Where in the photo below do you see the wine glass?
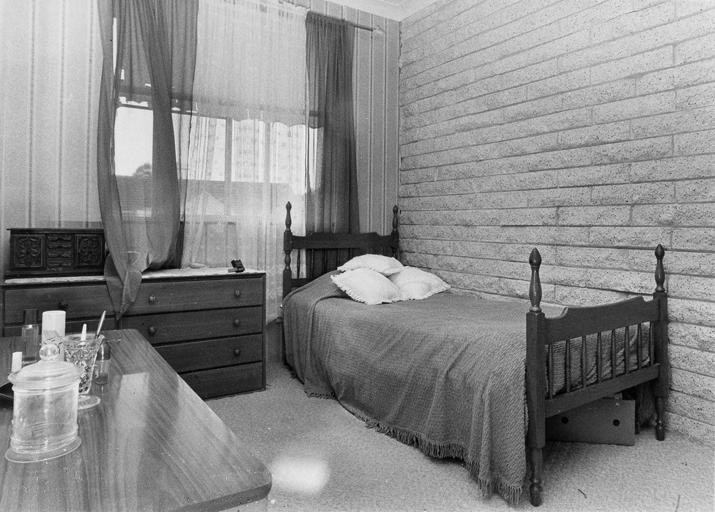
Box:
[61,332,103,411]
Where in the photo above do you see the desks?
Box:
[0,330,273,512]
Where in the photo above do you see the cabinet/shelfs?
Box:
[3,266,273,402]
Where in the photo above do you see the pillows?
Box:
[331,253,450,306]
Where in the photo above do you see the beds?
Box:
[281,199,672,508]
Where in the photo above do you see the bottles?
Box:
[3,343,83,464]
[40,309,68,360]
[93,338,111,385]
[20,307,41,364]
